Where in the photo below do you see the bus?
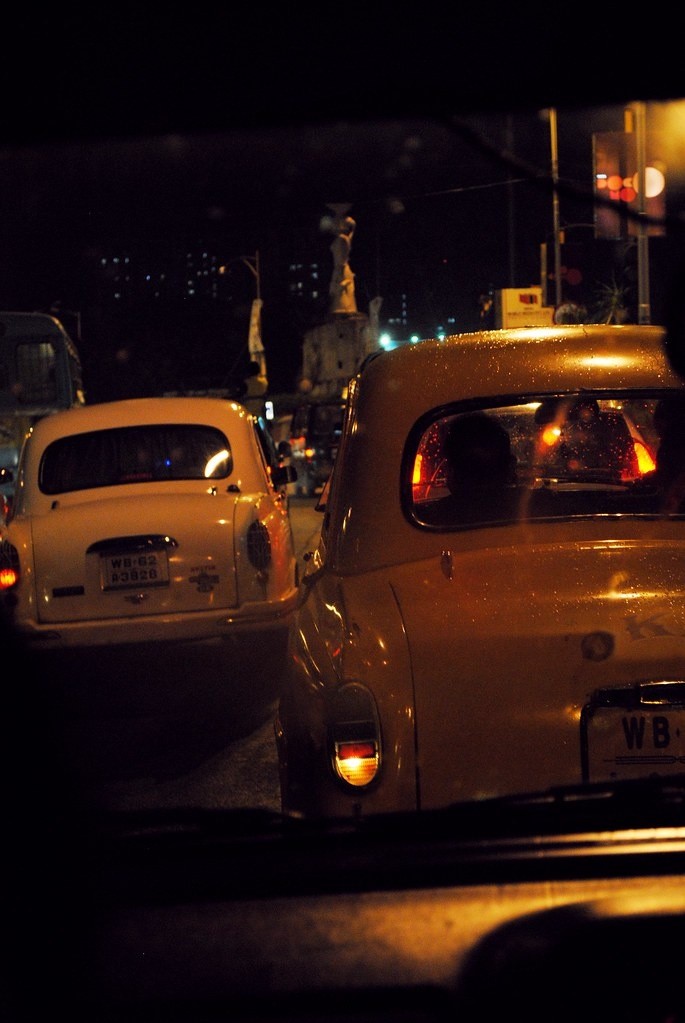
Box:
[0,312,85,479]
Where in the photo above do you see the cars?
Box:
[412,403,661,499]
[279,322,683,812]
[0,400,306,655]
[284,400,350,494]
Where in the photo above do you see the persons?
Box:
[73,436,208,476]
[427,413,545,517]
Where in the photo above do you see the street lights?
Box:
[539,106,566,311]
[217,250,267,378]
